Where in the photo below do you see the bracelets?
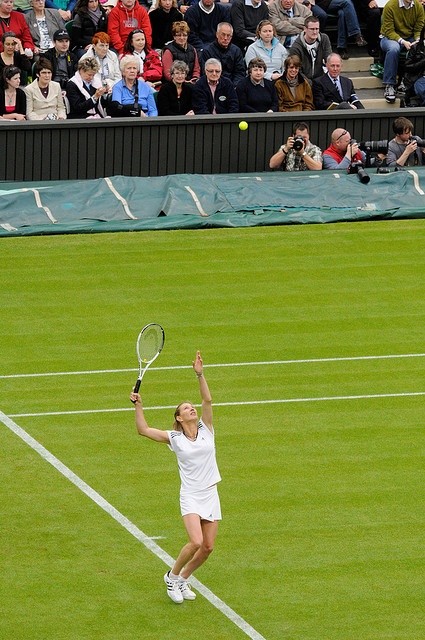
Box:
[195,373,203,377]
[282,148,288,155]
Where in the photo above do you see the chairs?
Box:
[63,21,74,28]
[31,63,38,75]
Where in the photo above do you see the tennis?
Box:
[239,121,248,130]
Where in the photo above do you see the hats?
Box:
[54,29,70,40]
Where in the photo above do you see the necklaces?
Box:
[5,89,16,106]
[39,84,49,94]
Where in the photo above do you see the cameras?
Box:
[409,135,425,148]
[347,162,371,184]
[293,135,305,155]
[346,139,389,156]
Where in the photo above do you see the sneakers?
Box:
[339,48,349,59]
[397,84,407,94]
[176,580,197,601]
[354,36,368,47]
[384,86,396,103]
[163,570,185,603]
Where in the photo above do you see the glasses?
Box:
[337,129,349,140]
[306,27,319,30]
[175,34,187,37]
[40,71,53,74]
[173,72,186,75]
[206,68,221,73]
[132,28,144,33]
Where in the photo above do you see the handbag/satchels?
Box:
[121,102,143,118]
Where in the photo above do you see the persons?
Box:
[45,0,79,22]
[25,57,67,120]
[298,0,329,32]
[380,0,425,104]
[157,59,198,115]
[119,29,163,81]
[386,117,425,167]
[268,122,323,172]
[317,1,368,60]
[136,0,157,13]
[97,0,120,16]
[0,65,27,120]
[71,0,119,58]
[162,21,201,85]
[274,55,316,111]
[404,26,425,107]
[353,0,381,59]
[232,0,269,45]
[288,15,333,80]
[178,0,199,15]
[184,1,232,53]
[65,59,113,118]
[243,19,289,81]
[313,52,361,109]
[107,0,153,59]
[237,58,279,112]
[322,127,366,170]
[269,0,313,36]
[0,0,35,60]
[40,30,78,88]
[199,22,246,89]
[192,57,240,113]
[25,0,68,55]
[78,31,124,100]
[128,350,222,606]
[148,0,184,51]
[0,31,31,86]
[111,54,158,116]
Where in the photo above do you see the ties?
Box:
[286,11,291,47]
[334,79,340,96]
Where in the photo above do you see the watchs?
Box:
[299,152,307,158]
